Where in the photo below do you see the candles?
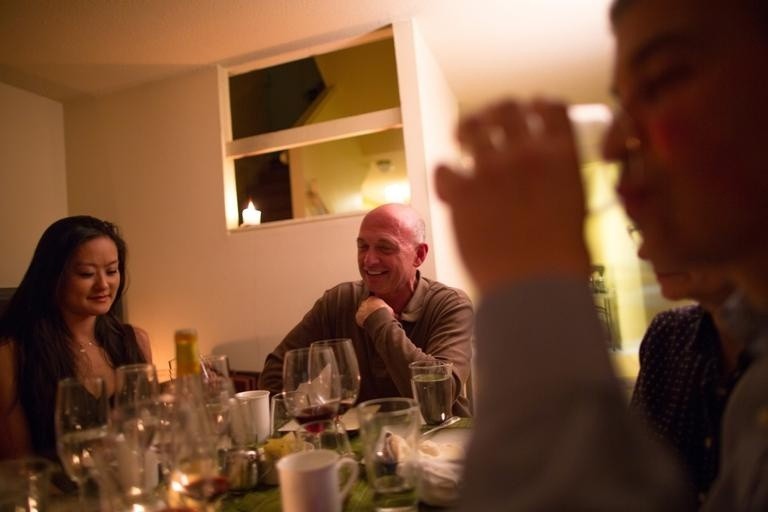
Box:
[240,200,260,224]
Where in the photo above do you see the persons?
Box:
[0,215,157,498]
[256,201,476,421]
[433,0,768,510]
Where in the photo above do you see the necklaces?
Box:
[64,332,99,353]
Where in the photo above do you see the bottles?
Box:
[171,327,209,414]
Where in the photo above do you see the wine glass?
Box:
[54,337,362,511]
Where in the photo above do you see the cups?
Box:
[354,397,422,511]
[434,95,646,218]
[409,358,454,425]
[1,456,54,512]
